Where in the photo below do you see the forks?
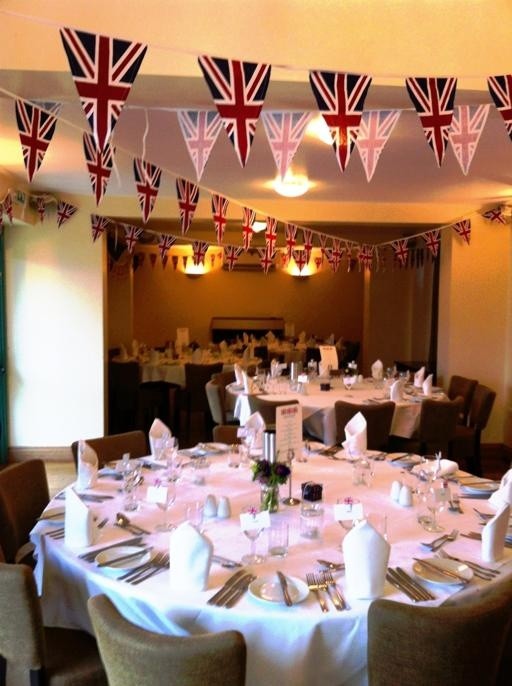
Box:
[118,553,171,584]
[305,569,346,613]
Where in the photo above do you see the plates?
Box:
[247,576,309,604]
[95,546,150,569]
[413,559,474,586]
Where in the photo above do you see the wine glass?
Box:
[38,426,512,561]
[251,359,412,399]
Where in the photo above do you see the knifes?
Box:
[207,568,255,610]
[412,558,467,584]
[276,571,294,607]
[386,566,432,604]
